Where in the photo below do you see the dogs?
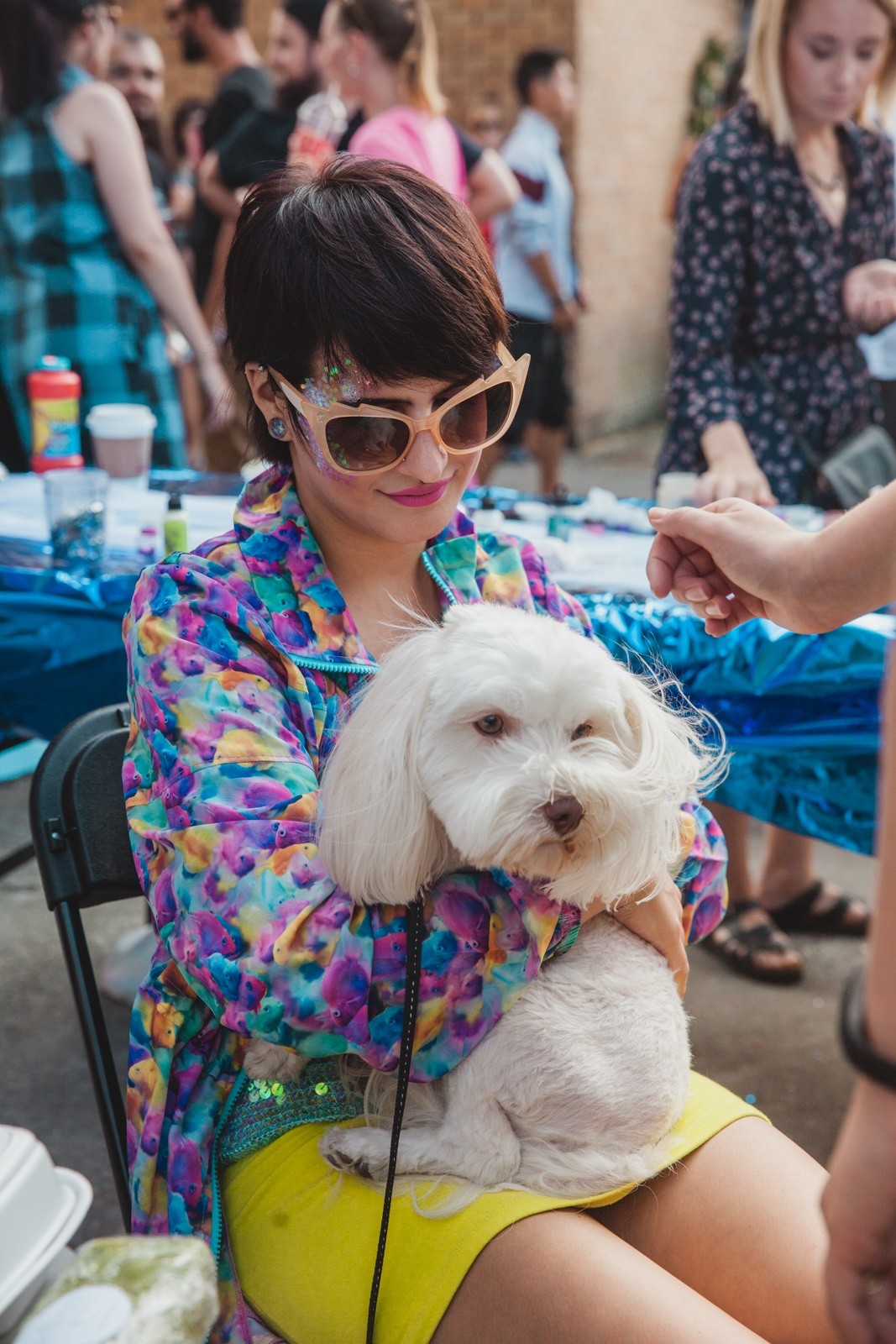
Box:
[302,587,730,1221]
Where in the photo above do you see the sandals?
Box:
[771,876,871,938]
[697,896,802,986]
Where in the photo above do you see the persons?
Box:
[0,0,896,1344]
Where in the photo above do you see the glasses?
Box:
[262,334,532,478]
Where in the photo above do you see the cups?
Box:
[43,467,111,583]
[85,403,157,480]
[286,91,348,183]
[657,472,696,509]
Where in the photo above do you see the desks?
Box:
[0,467,896,883]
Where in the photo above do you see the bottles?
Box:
[29,353,83,474]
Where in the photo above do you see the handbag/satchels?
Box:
[820,422,896,511]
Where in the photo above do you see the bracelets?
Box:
[834,962,896,1094]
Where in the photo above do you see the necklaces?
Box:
[807,167,842,194]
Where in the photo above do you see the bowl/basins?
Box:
[0,1121,93,1336]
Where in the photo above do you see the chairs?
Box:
[26,700,151,1224]
